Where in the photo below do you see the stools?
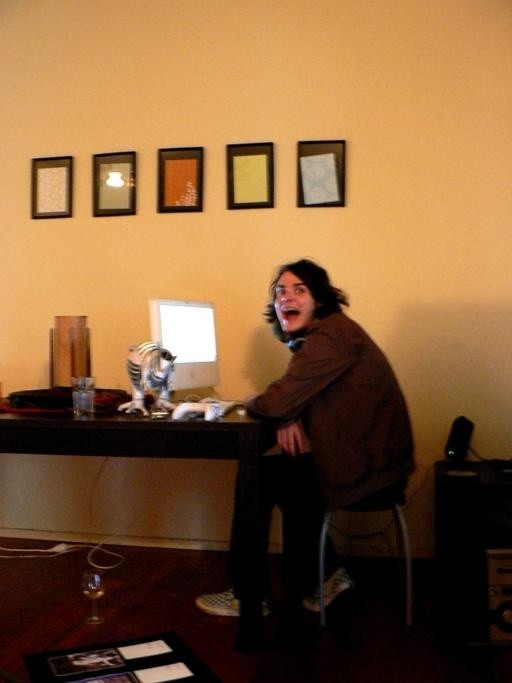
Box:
[313,493,414,629]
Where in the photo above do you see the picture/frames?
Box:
[226,142,275,211]
[157,146,204,213]
[296,139,346,208]
[92,150,137,217]
[31,155,73,221]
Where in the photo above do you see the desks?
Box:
[0,387,310,556]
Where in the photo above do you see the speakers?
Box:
[444,415,475,459]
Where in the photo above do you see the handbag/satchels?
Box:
[0,386,127,415]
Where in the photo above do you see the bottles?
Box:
[72,377,96,420]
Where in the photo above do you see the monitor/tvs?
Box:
[149,299,220,410]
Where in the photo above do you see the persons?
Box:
[197,260,415,617]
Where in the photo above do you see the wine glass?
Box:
[81,569,105,625]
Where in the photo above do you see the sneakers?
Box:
[196,588,269,617]
[304,567,354,611]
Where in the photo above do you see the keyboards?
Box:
[198,397,237,417]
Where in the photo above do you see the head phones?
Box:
[288,300,335,353]
